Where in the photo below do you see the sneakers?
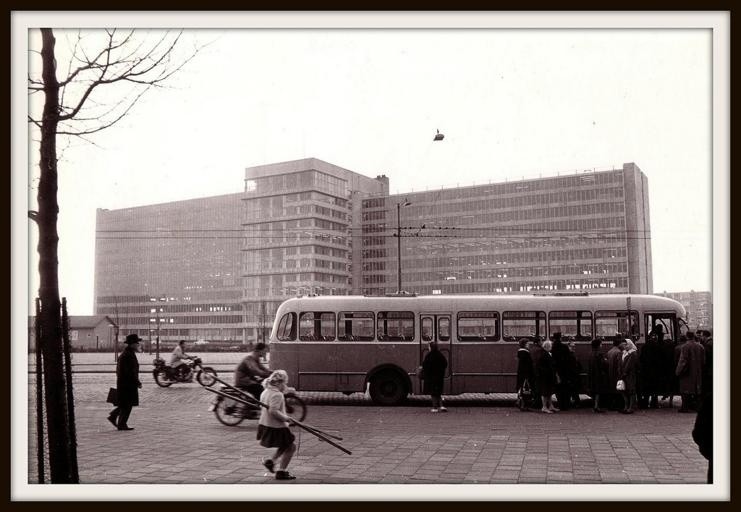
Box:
[264,460,275,473]
[430,406,447,412]
[106,415,134,430]
[540,404,559,414]
[276,471,296,480]
[592,404,632,414]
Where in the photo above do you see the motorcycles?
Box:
[206,380,308,427]
[150,355,219,388]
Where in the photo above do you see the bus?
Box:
[260,295,700,408]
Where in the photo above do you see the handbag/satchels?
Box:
[518,380,534,409]
[616,379,625,390]
[107,387,118,406]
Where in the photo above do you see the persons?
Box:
[255,370,297,480]
[421,341,448,413]
[234,342,272,402]
[692,392,712,483]
[107,332,143,431]
[516,324,712,414]
[169,340,193,368]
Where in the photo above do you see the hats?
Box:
[124,334,142,343]
